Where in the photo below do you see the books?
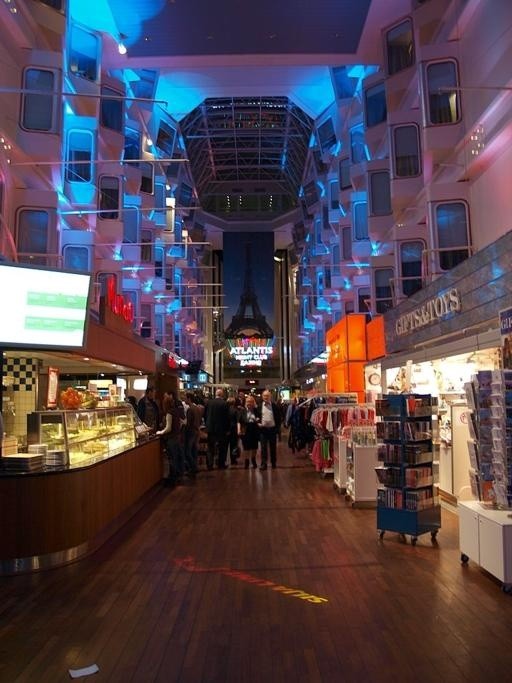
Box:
[462,367,511,505]
[373,393,442,514]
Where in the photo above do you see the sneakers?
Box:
[185,473,196,480]
[176,478,182,483]
[191,468,198,472]
[164,481,174,487]
[206,458,277,470]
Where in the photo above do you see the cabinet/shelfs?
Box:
[439,445,452,494]
[377,394,442,547]
[25,403,136,465]
[457,370,512,592]
[346,438,384,509]
[289,392,377,476]
[450,402,472,494]
[332,434,347,494]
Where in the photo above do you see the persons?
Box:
[124,385,317,485]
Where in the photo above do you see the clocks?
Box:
[369,373,381,384]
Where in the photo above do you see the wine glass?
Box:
[110,395,120,408]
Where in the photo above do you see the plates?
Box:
[53,434,79,441]
[46,450,68,470]
[27,445,51,470]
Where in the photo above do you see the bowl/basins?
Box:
[57,399,84,411]
[82,398,100,411]
